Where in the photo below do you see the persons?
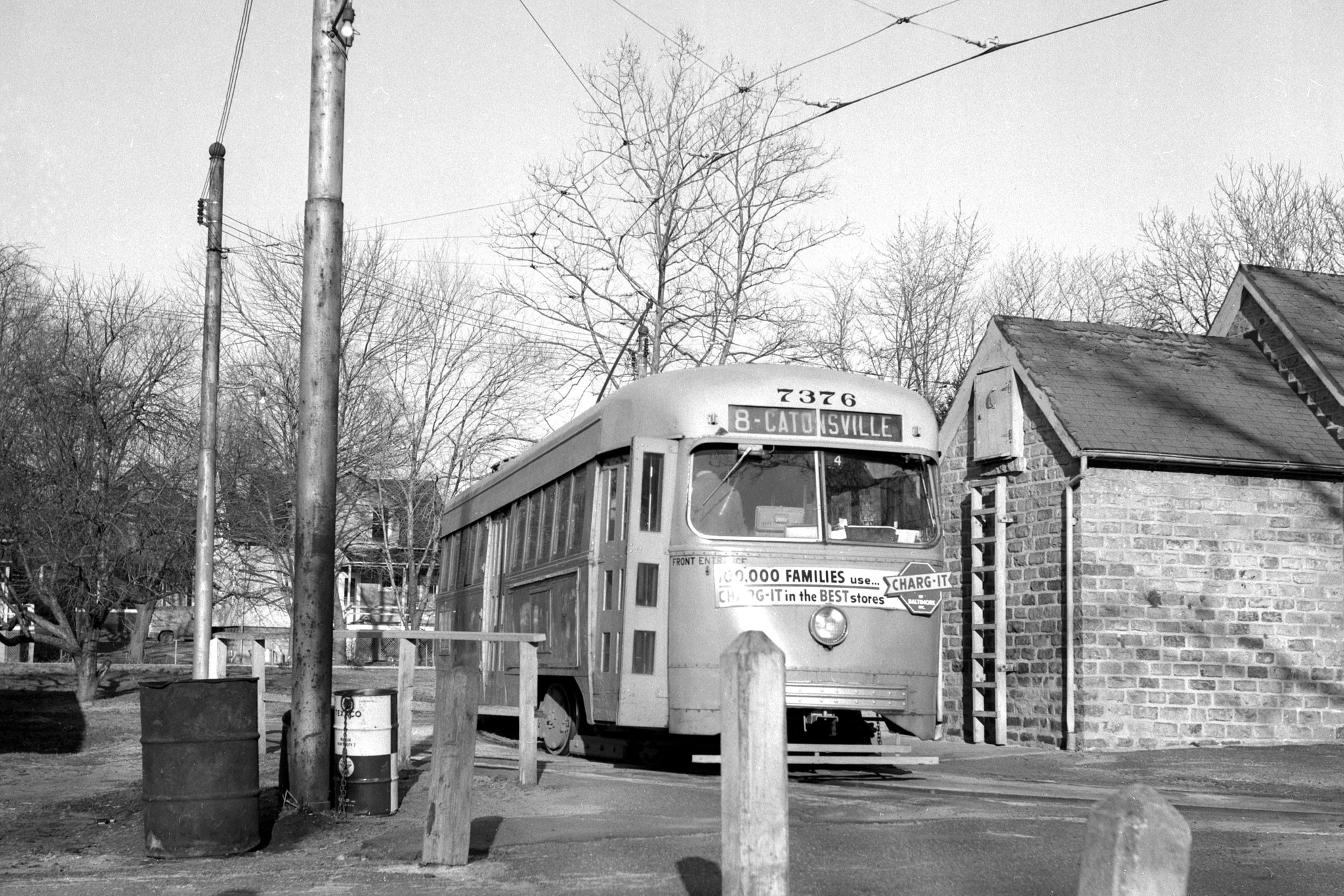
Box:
[689,451,747,539]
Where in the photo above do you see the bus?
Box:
[440,362,947,780]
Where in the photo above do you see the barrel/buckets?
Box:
[139,674,263,858]
[335,686,401,816]
[278,703,336,807]
